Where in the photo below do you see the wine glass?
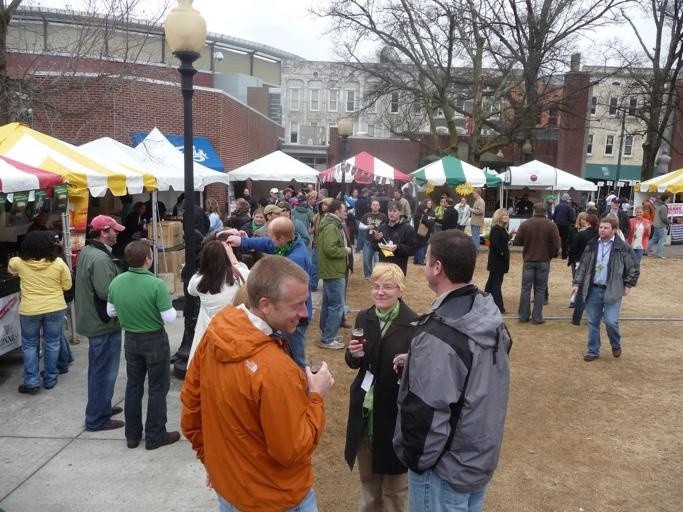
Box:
[310,359,321,374]
[352,328,363,357]
[394,354,406,384]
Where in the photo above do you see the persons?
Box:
[391,228,514,512]
[179,254,336,512]
[342,260,420,512]
[187,175,518,370]
[515,190,671,362]
[7,193,184,451]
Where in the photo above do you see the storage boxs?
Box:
[158,250,185,292]
[148,220,184,249]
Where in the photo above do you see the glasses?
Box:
[371,285,400,290]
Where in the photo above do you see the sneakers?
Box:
[320,341,345,349]
[612,347,622,358]
[584,352,599,361]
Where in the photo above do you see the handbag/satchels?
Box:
[417,215,428,237]
[665,223,671,246]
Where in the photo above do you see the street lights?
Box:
[521,137,534,163]
[337,118,354,199]
[162,0,208,379]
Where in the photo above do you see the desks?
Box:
[465,217,528,241]
[0,290,21,356]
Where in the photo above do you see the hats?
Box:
[612,198,621,203]
[270,188,278,193]
[90,214,126,232]
[264,205,286,215]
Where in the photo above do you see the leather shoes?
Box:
[19,384,40,393]
[109,407,123,415]
[145,431,180,449]
[105,420,124,430]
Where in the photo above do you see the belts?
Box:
[593,284,607,288]
